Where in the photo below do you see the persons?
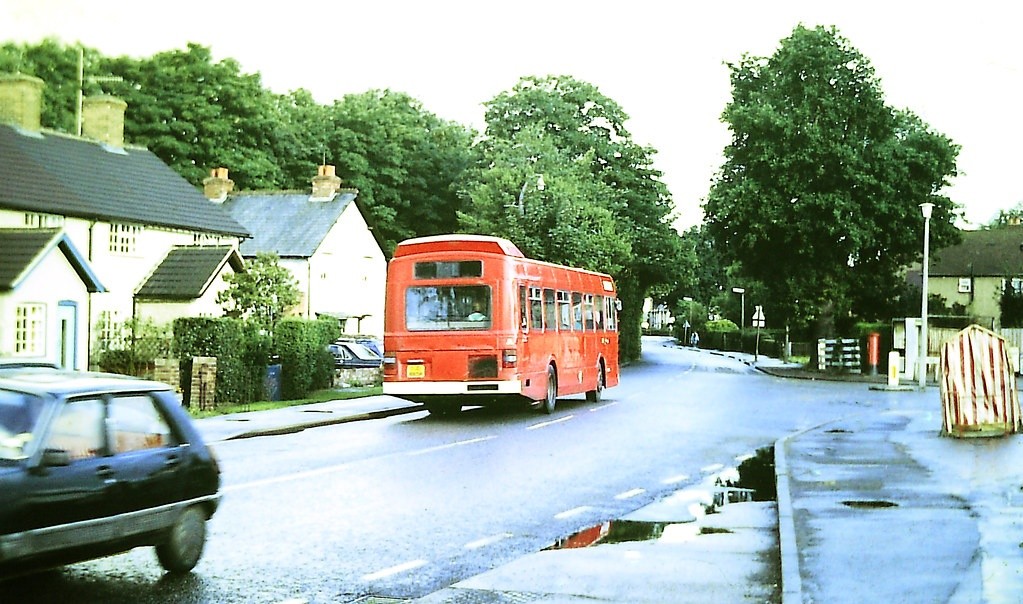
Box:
[690,331,699,348]
[468,303,487,321]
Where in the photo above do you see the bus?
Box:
[382,234,623,415]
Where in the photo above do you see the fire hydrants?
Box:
[867,332,880,375]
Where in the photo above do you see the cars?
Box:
[326,342,383,369]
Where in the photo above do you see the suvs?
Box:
[0,363,223,572]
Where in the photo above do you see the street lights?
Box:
[733,287,745,350]
[519,174,544,216]
[918,203,935,388]
[683,297,692,344]
